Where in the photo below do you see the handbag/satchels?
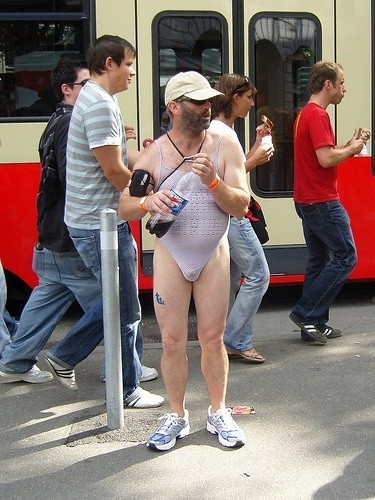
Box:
[251,217,269,244]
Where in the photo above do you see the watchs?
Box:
[139,196,148,212]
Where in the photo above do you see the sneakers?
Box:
[0,363,53,383]
[288,312,327,344]
[206,405,246,448]
[146,409,191,451]
[103,386,165,408]
[301,324,342,341]
[44,355,79,392]
[100,363,158,382]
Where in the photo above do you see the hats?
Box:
[165,71,225,107]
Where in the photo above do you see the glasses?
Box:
[174,97,214,105]
[232,76,250,94]
[66,79,88,87]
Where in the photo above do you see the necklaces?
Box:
[166,130,206,159]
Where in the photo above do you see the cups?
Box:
[356,128,370,142]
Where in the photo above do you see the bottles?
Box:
[144,172,201,238]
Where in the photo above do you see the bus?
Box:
[0,0,375,294]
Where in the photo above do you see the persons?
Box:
[43,35,163,408]
[0,60,157,383]
[119,70,250,450]
[0,259,19,360]
[205,74,274,363]
[288,61,369,343]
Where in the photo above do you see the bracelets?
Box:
[206,174,220,190]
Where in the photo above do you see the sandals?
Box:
[225,345,265,362]
